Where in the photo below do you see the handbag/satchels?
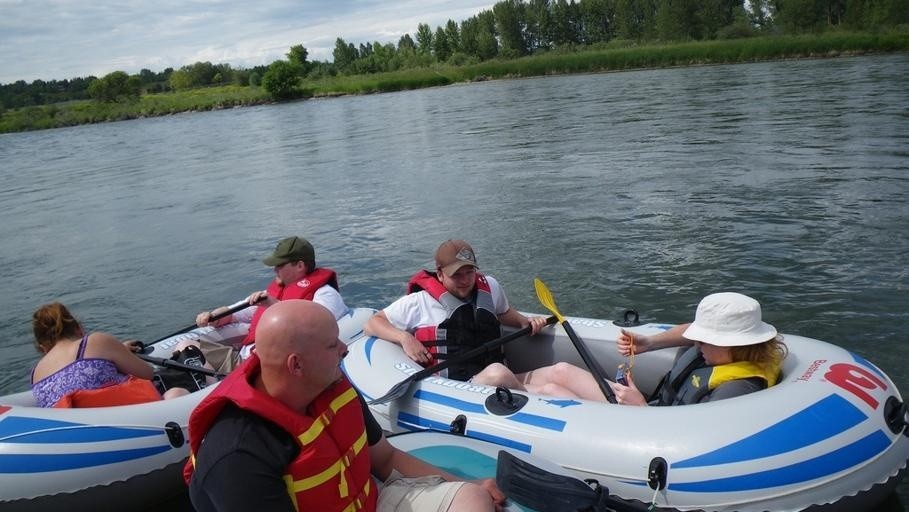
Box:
[152,345,205,394]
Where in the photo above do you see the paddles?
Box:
[365,315,559,404]
[533,278,617,404]
[130,294,268,351]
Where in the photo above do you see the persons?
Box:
[514,292,788,407]
[176,235,348,387]
[363,238,547,392]
[183,298,507,512]
[29,302,192,410]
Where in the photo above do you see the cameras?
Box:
[617,362,631,386]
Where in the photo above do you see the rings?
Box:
[424,351,429,356]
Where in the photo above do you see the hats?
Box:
[263,236,314,266]
[682,292,777,346]
[436,240,479,277]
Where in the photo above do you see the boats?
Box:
[341,292,899,510]
[3,296,377,511]
